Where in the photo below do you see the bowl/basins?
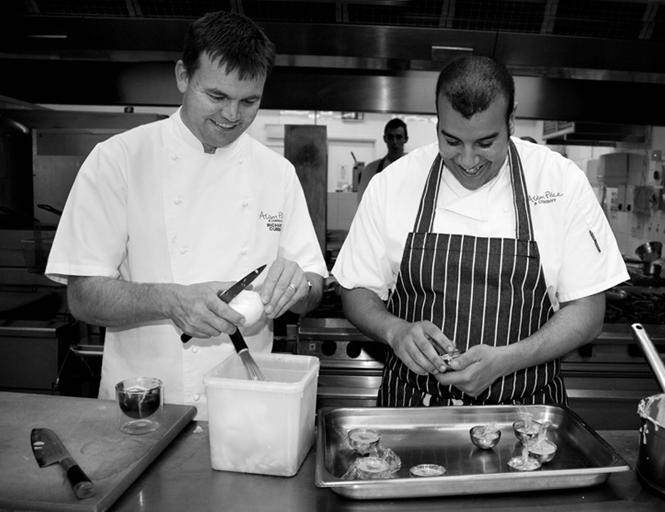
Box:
[643,264,662,275]
[635,241,663,263]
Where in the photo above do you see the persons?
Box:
[356,117,409,207]
[43,5,332,423]
[328,50,631,410]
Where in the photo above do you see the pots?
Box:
[628,320,665,500]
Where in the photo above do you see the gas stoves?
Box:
[562,288,665,360]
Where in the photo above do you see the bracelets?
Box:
[307,277,314,295]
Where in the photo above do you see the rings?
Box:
[288,282,300,294]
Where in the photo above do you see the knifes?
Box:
[29,427,96,501]
[180,265,267,345]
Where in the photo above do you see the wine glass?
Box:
[114,378,164,435]
